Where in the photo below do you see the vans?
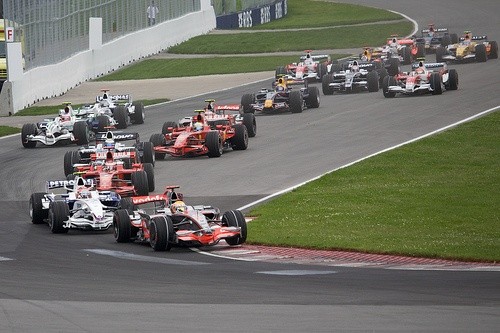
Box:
[0,20,27,82]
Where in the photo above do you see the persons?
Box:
[361,56,368,62]
[193,122,203,131]
[171,201,188,214]
[462,40,470,46]
[390,43,397,48]
[428,32,434,37]
[304,60,312,65]
[275,84,285,92]
[205,110,214,118]
[351,66,359,72]
[61,113,71,121]
[73,190,92,213]
[415,68,424,74]
[103,139,115,149]
[101,99,110,107]
[104,160,117,173]
[146,0,159,26]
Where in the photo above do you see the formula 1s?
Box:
[114,185,249,253]
[275,22,459,96]
[20,87,257,233]
[382,58,459,98]
[434,29,498,65]
[240,74,321,114]
